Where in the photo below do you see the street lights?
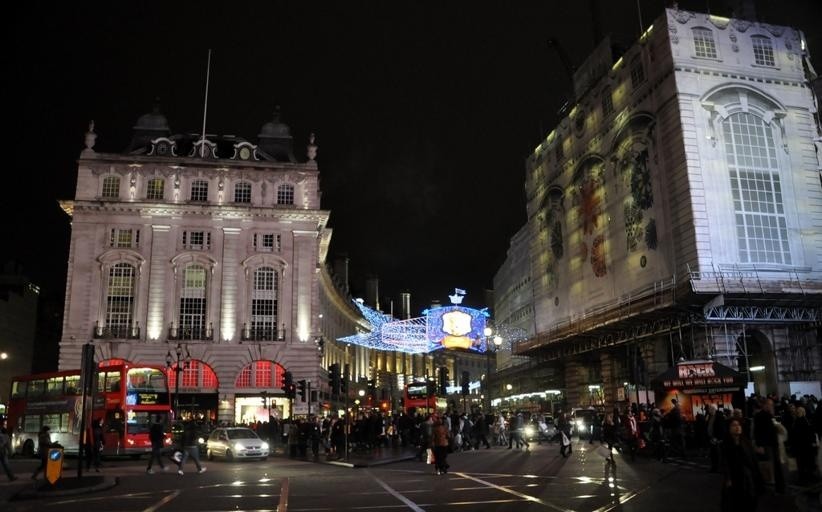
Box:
[476,319,503,415]
[166,343,191,419]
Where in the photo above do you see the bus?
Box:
[404,382,436,417]
[6,363,173,460]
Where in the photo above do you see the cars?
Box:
[171,419,216,453]
[205,426,270,463]
[485,407,604,443]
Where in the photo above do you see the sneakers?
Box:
[177,469,185,475]
[433,466,447,476]
[198,466,207,474]
[160,464,169,473]
[145,468,155,475]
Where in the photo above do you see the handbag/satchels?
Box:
[169,449,183,465]
[426,447,437,465]
[96,441,104,452]
[438,438,449,446]
[596,445,612,457]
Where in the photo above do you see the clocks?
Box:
[157,143,169,155]
[199,145,210,157]
[239,147,250,160]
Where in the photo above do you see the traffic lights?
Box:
[297,379,306,402]
[463,371,470,396]
[441,367,449,395]
[260,390,267,408]
[341,375,348,392]
[288,385,296,398]
[281,371,291,397]
[327,362,340,395]
[367,379,376,399]
[429,380,436,398]
[271,399,276,409]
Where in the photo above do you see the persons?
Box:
[430,419,450,475]
[145,415,202,475]
[0,426,19,482]
[253,413,576,457]
[86,417,104,473]
[31,426,51,481]
[601,394,822,489]
